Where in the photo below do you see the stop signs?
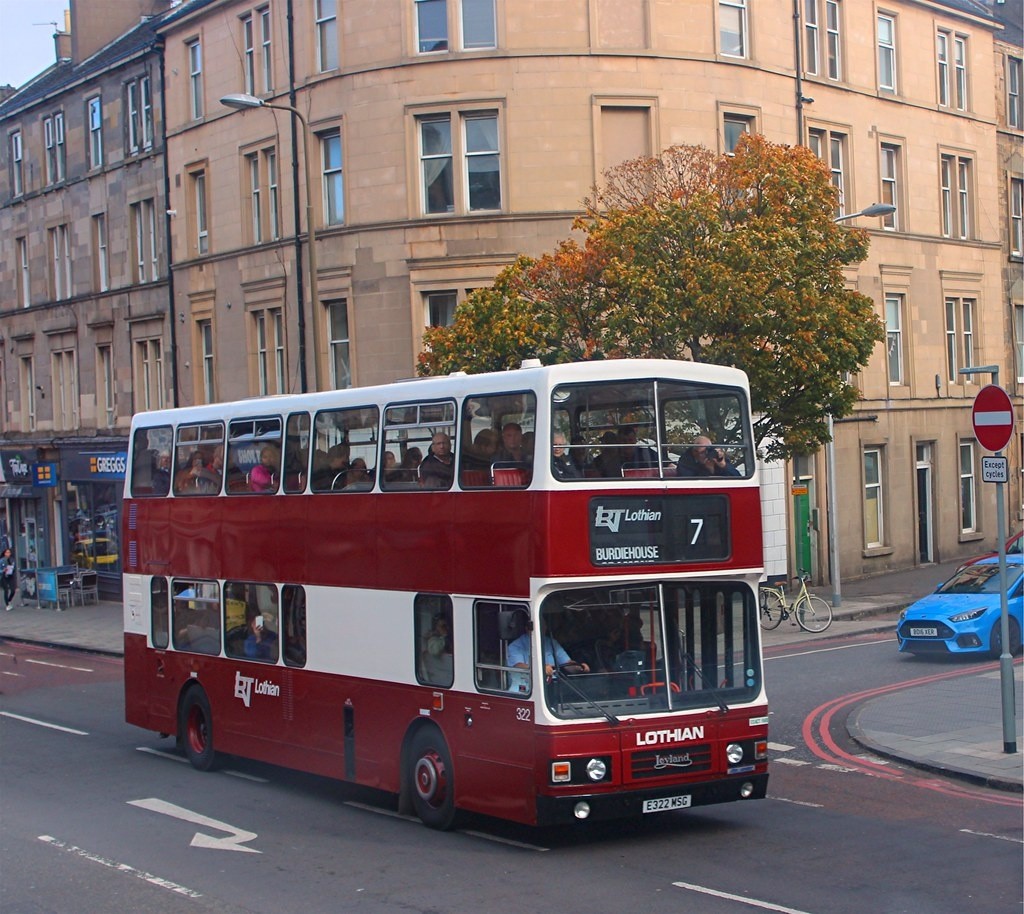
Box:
[970,385,1015,454]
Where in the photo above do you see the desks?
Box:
[20,567,76,576]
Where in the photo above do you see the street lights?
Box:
[219,93,324,393]
[826,204,896,606]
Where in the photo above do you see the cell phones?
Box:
[196,459,202,466]
[256,616,264,630]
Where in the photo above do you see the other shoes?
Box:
[8,602,14,609]
[6,606,11,611]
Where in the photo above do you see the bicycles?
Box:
[759,568,834,633]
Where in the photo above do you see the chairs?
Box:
[35,569,100,611]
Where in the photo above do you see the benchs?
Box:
[583,461,607,477]
[621,460,679,477]
[491,460,533,486]
[227,474,251,492]
[246,473,252,492]
[331,468,419,491]
[271,470,300,490]
[417,464,490,487]
[298,471,306,490]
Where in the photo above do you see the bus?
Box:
[121,359,769,830]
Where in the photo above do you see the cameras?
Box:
[705,447,723,462]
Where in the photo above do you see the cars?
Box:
[896,531,1024,659]
[68,504,119,570]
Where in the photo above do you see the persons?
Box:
[676,436,742,476]
[386,448,422,483]
[488,423,534,470]
[469,428,497,469]
[0,549,17,611]
[553,433,579,479]
[242,617,278,662]
[426,613,452,685]
[591,432,616,478]
[549,610,585,658]
[606,427,677,478]
[506,619,590,696]
[205,445,233,476]
[419,432,454,487]
[604,625,624,662]
[249,443,369,494]
[368,452,395,482]
[175,451,219,494]
